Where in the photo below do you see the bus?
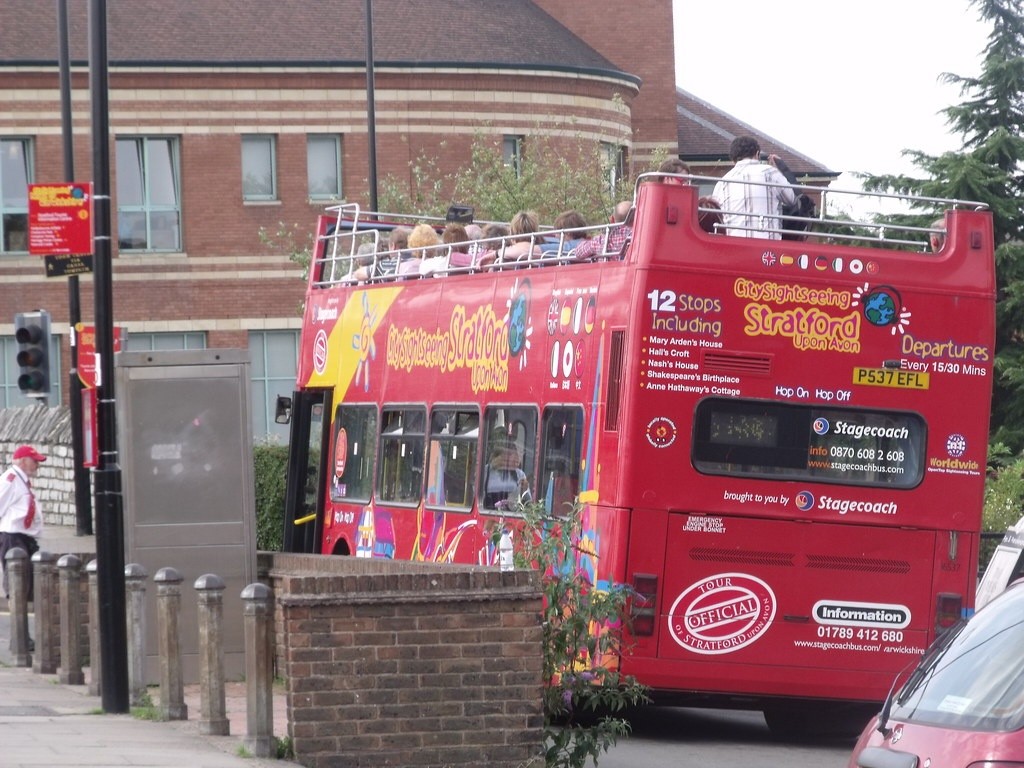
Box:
[271,168,998,743]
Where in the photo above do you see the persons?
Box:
[0,446,47,600]
[338,153,815,286]
[482,426,533,514]
[711,136,795,240]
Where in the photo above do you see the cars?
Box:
[848,518,1024,768]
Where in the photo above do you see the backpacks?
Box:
[774,157,817,242]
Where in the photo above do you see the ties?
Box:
[24,479,36,529]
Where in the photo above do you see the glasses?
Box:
[674,177,688,186]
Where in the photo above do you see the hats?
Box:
[13,445,47,461]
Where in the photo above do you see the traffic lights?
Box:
[16,326,47,392]
